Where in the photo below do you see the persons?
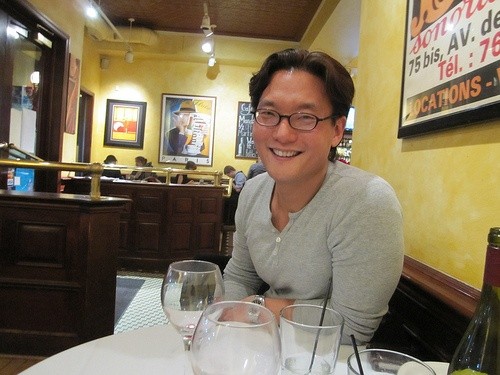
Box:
[101,155,124,179]
[21,86,36,108]
[212,48,404,347]
[129,156,158,183]
[224,165,266,226]
[177,161,197,184]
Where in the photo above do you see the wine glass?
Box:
[160,260,225,374]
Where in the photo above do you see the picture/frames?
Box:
[159,93,216,166]
[235,101,259,159]
[104,99,147,150]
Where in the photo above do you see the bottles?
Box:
[448,226,500,375]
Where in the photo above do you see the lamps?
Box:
[200,0,216,65]
[124,17,135,63]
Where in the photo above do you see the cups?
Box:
[278,304,344,374]
[347,348,436,375]
[190,300,278,374]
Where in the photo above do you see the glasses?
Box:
[250,108,338,131]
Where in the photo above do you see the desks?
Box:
[15,327,398,375]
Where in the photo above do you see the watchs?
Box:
[246,295,265,324]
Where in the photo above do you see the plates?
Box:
[398,360,450,374]
